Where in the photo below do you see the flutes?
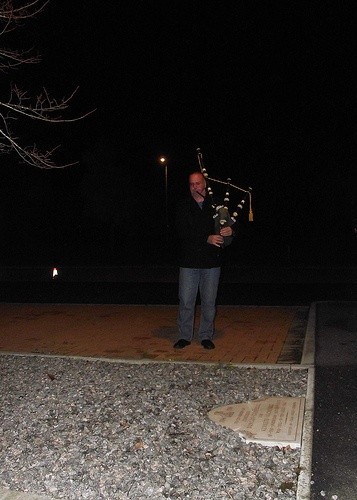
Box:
[194,147,256,258]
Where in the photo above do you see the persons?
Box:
[173,171,233,350]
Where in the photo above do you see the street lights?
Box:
[159,156,169,228]
